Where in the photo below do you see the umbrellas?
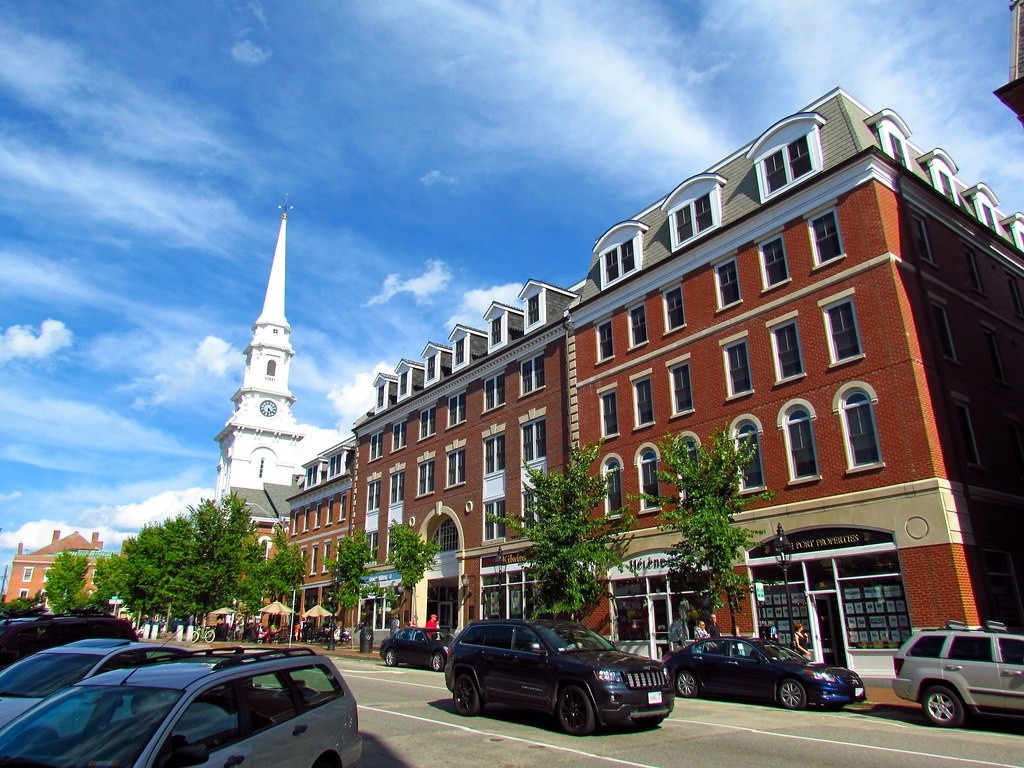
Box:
[210,607,235,614]
[258,600,296,615]
[302,605,332,627]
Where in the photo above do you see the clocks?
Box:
[260,400,278,417]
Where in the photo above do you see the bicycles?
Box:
[192,626,216,643]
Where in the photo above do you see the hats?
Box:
[431,614,437,618]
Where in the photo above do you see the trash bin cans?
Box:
[359,627,373,653]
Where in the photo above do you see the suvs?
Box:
[0,610,147,671]
[0,644,363,768]
[444,618,681,737]
[891,619,1024,728]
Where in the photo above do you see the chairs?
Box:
[174,702,232,744]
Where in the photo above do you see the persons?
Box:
[216,613,338,651]
[793,623,812,660]
[426,614,441,640]
[669,614,722,659]
[390,619,399,636]
[406,615,418,628]
[125,615,165,633]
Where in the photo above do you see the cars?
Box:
[661,635,869,710]
[0,638,206,751]
[379,627,461,672]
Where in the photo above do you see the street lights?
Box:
[772,522,798,652]
[329,567,342,651]
[246,501,296,648]
[494,545,508,619]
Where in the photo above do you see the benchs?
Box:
[202,692,297,735]
